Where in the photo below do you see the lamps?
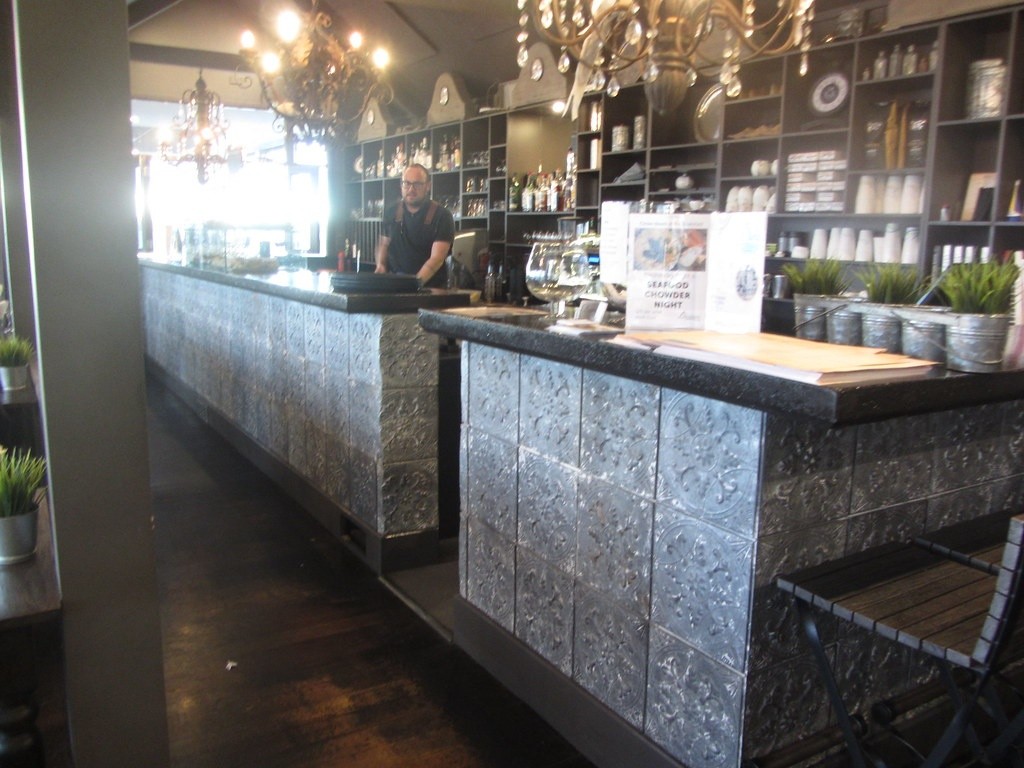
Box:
[229,0,395,145]
[515,0,817,115]
[159,68,231,185]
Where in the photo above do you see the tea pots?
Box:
[676,173,694,189]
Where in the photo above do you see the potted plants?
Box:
[0,333,37,392]
[782,251,856,341]
[850,257,1024,373]
[0,444,48,556]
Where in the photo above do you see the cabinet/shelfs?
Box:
[340,1,1024,337]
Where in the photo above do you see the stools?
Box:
[775,505,1024,768]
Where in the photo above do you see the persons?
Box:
[373,164,455,288]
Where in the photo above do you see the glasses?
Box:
[399,179,427,190]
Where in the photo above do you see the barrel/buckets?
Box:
[794,294,826,340]
[826,302,862,345]
[900,306,951,361]
[945,312,1009,373]
[861,308,900,353]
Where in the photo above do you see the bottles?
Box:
[410,137,432,170]
[580,217,600,254]
[524,232,573,244]
[173,227,225,269]
[466,174,488,217]
[436,135,459,170]
[436,193,459,218]
[862,41,937,81]
[466,151,488,167]
[368,197,383,218]
[486,252,503,304]
[377,150,383,177]
[509,148,575,213]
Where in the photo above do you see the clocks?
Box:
[801,70,851,131]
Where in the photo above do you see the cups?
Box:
[772,275,791,299]
[779,223,920,265]
[855,176,924,215]
[726,157,777,212]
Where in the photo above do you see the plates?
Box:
[807,72,850,117]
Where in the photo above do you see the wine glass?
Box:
[525,243,590,322]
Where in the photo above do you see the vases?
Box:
[823,295,863,346]
